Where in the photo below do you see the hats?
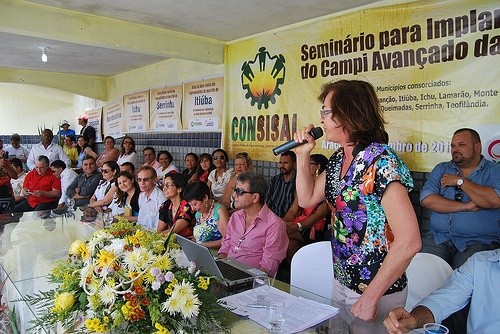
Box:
[42,133,51,137]
[78,114,88,120]
[60,120,70,128]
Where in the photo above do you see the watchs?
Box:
[456,176,466,188]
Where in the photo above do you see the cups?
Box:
[424,323,449,334]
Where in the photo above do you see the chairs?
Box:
[290,241,332,299]
[405,253,454,333]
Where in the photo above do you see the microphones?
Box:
[273,127,323,156]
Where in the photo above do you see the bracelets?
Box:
[297,223,303,232]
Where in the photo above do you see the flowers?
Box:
[7,214,250,334]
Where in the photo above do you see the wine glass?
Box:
[104,212,113,228]
[266,301,285,334]
[253,276,270,306]
[67,198,75,211]
[67,210,75,221]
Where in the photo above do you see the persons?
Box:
[57,120,76,149]
[420,128,499,271]
[295,80,423,334]
[0,129,139,216]
[283,154,332,284]
[136,166,168,230]
[183,180,229,258]
[214,172,289,278]
[138,146,161,171]
[216,152,254,217]
[115,172,141,225]
[206,149,234,202]
[383,249,500,334]
[266,150,297,219]
[157,173,197,241]
[75,115,96,156]
[181,152,199,184]
[188,153,216,183]
[155,150,182,190]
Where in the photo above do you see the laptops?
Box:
[175,234,268,287]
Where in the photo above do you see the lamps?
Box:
[39,47,49,63]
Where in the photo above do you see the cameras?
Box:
[27,192,33,194]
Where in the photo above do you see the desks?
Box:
[0,209,422,334]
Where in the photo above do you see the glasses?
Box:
[123,141,133,144]
[455,185,462,202]
[137,177,154,182]
[161,182,176,189]
[233,187,256,196]
[213,156,224,160]
[320,110,332,115]
[101,169,114,173]
[279,161,293,164]
[52,171,56,173]
